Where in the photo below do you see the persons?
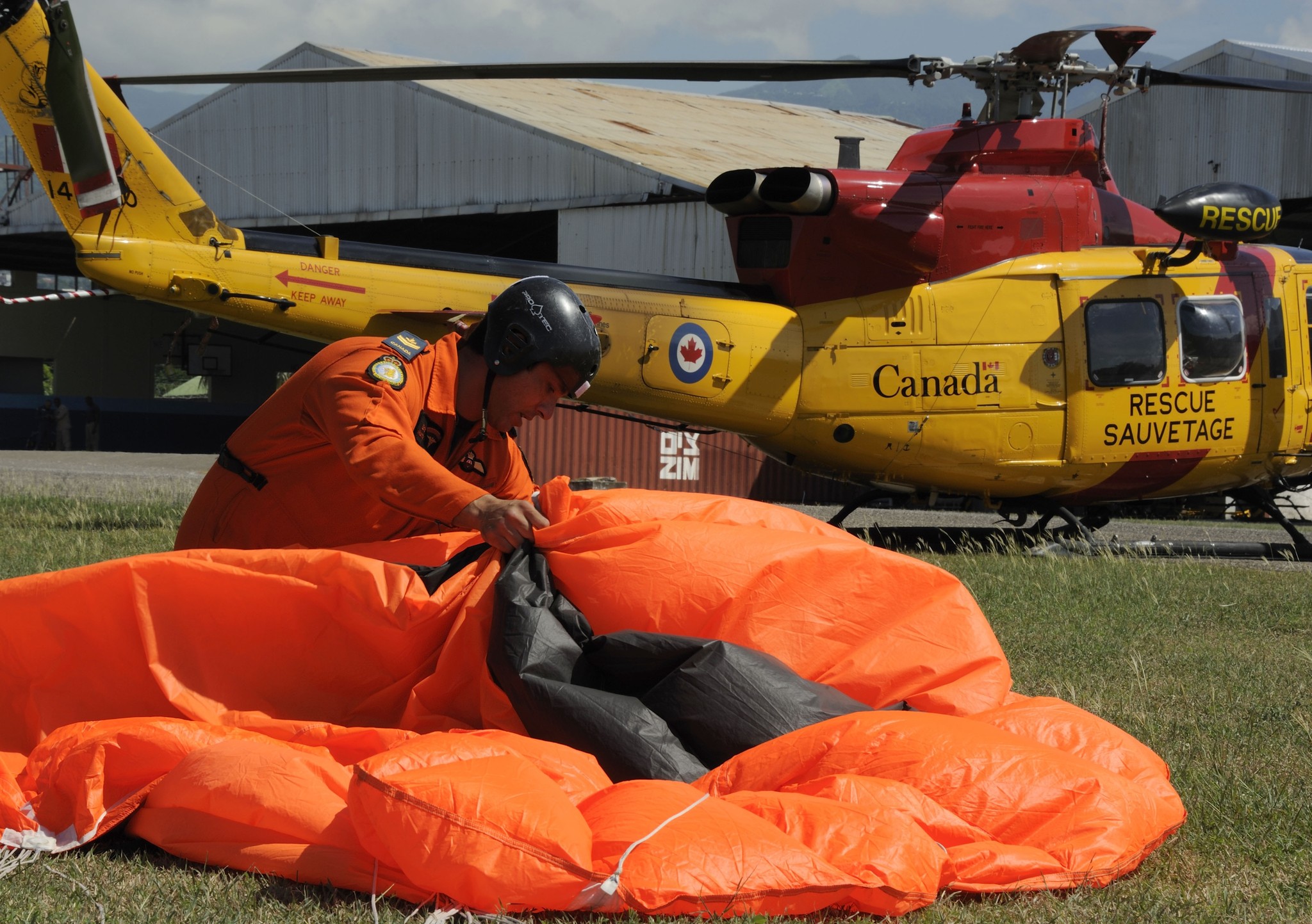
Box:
[174,278,601,555]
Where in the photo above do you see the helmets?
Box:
[483,276,602,399]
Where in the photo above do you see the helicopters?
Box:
[1,1,1312,554]
